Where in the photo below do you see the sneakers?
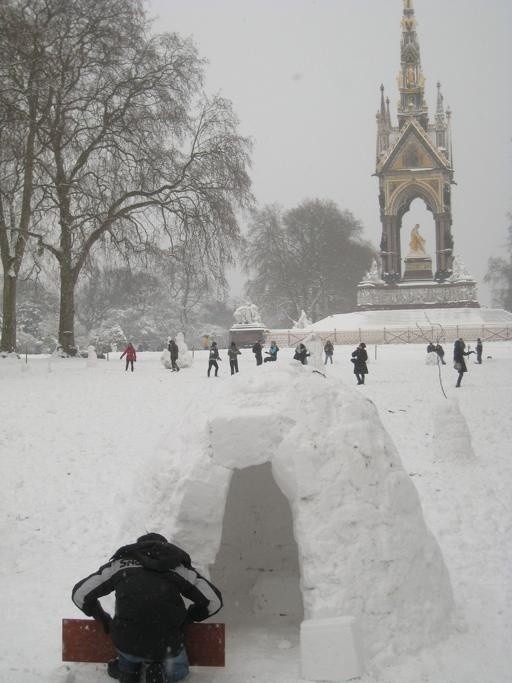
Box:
[146,662,191,683]
[107,658,141,682]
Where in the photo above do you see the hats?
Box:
[360,342,365,347]
[231,341,236,347]
[212,341,216,345]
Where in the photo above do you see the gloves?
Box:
[94,601,113,635]
[184,610,194,626]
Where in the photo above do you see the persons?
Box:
[207,341,221,376]
[120,342,137,371]
[264,339,279,362]
[435,343,446,364]
[476,337,482,364]
[228,343,241,374]
[168,340,180,372]
[351,342,368,385]
[294,343,307,365]
[323,339,334,364]
[71,532,223,681]
[426,342,436,352]
[252,337,266,366]
[295,346,310,364]
[453,340,475,387]
[459,338,466,357]
[411,224,427,255]
